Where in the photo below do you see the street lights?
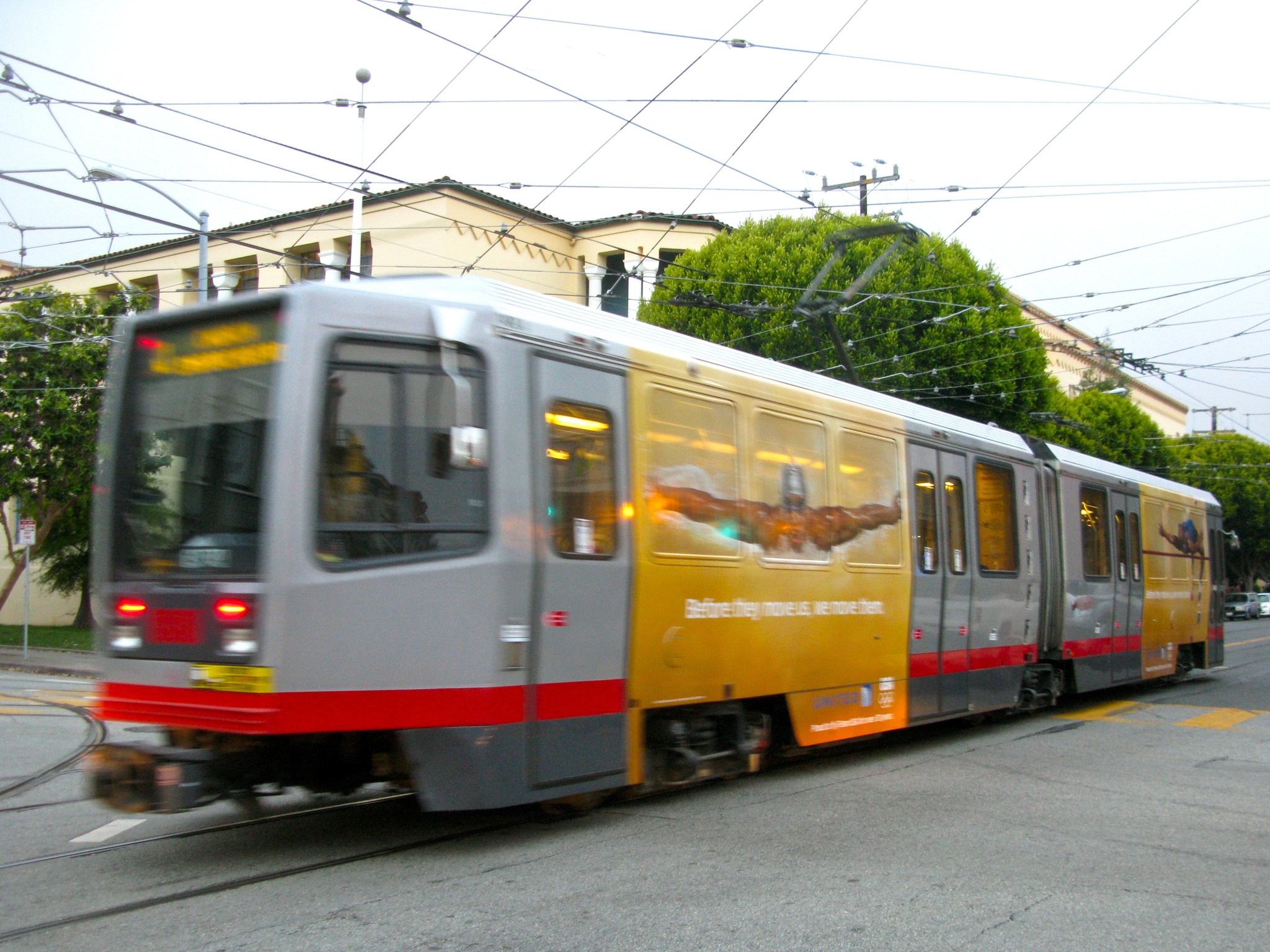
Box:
[89,167,219,313]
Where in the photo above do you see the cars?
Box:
[1258,593,1270,618]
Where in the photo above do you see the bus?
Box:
[78,222,1230,823]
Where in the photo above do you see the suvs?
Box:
[1224,592,1260,622]
